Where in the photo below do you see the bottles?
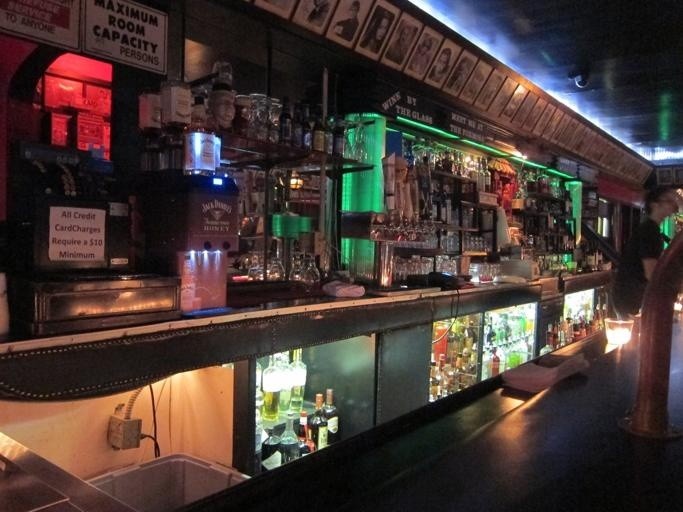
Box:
[462,348,471,389]
[470,346,477,384]
[527,218,543,246]
[260,426,281,471]
[308,393,326,451]
[430,191,447,225]
[440,359,446,398]
[280,99,292,148]
[301,104,312,151]
[445,356,454,396]
[333,116,344,158]
[546,304,608,348]
[483,305,536,378]
[290,347,307,413]
[322,387,338,444]
[454,352,465,392]
[557,220,574,251]
[252,359,262,430]
[431,318,479,360]
[290,104,302,147]
[311,116,323,152]
[429,360,437,402]
[297,411,310,457]
[279,412,299,461]
[433,149,491,192]
[323,116,332,155]
[262,354,282,428]
[580,239,603,270]
[281,350,290,412]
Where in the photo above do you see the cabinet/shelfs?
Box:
[342,112,582,286]
[138,72,375,287]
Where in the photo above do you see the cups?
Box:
[469,264,502,282]
[395,256,456,279]
[441,235,485,252]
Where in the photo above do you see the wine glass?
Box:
[248,257,319,282]
[370,225,435,248]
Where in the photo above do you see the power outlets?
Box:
[108,415,142,449]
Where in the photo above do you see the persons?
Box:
[385,26,413,65]
[428,48,452,83]
[359,10,393,55]
[606,186,677,321]
[408,38,431,76]
[332,0,360,42]
[305,0,332,28]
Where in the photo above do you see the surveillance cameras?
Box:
[575,72,588,89]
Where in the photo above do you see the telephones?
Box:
[429,272,465,293]
